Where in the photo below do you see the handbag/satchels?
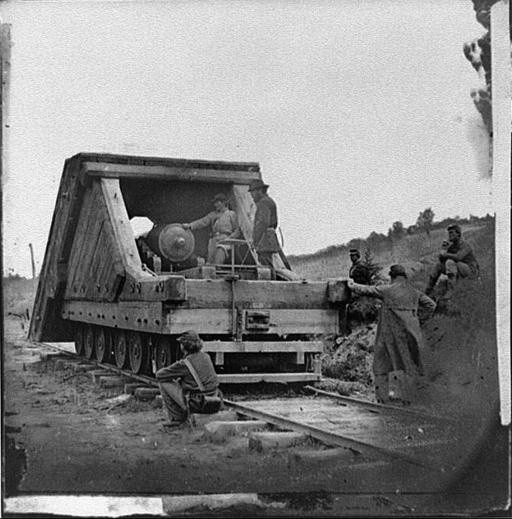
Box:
[202,396,221,413]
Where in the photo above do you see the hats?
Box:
[210,193,227,202]
[391,265,405,273]
[350,248,359,254]
[247,179,268,192]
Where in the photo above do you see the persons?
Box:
[246,177,281,281]
[348,247,371,297]
[422,224,481,300]
[346,264,437,407]
[181,191,241,273]
[156,329,224,428]
